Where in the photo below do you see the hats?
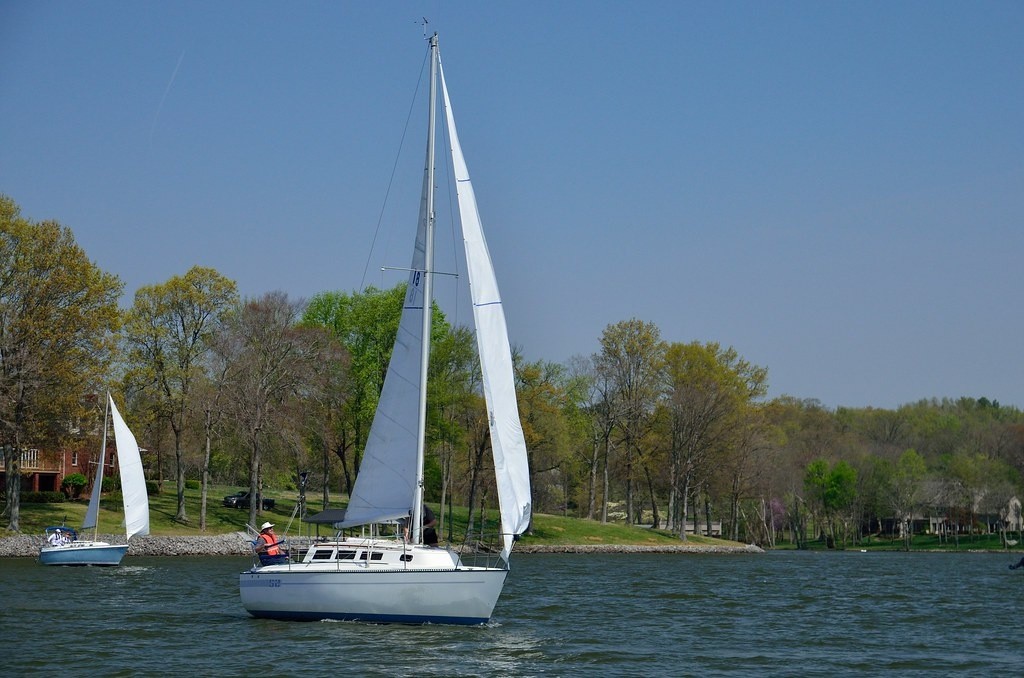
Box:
[261,522,275,532]
[55,529,60,532]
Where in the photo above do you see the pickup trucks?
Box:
[223,491,275,511]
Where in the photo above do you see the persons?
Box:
[404,505,438,548]
[47,529,71,547]
[255,522,289,566]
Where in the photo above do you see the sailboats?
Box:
[241,15,533,625]
[40,390,150,567]
